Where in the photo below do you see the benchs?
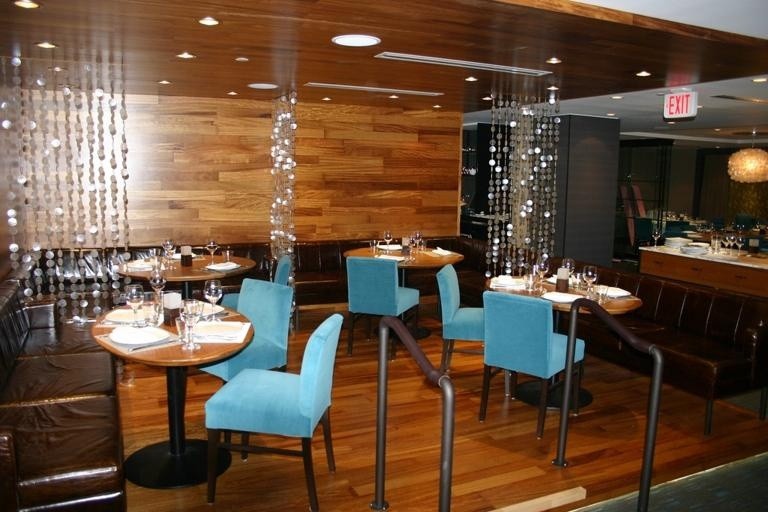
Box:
[111,238,462,312]
[465,238,766,434]
[0,245,127,512]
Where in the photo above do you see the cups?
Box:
[370,240,378,255]
[143,292,182,326]
[749,238,759,255]
[181,246,192,267]
[150,256,161,272]
[594,286,608,305]
[711,235,721,255]
[222,250,233,262]
[556,268,582,292]
[416,240,426,252]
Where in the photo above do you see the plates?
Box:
[105,308,159,324]
[206,262,240,271]
[598,286,632,299]
[377,245,402,251]
[540,291,584,304]
[664,237,694,249]
[182,302,224,318]
[111,327,170,345]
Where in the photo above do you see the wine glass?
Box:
[162,240,176,271]
[562,256,576,280]
[409,230,421,254]
[582,265,598,301]
[126,284,144,327]
[204,280,222,325]
[180,299,201,351]
[722,235,744,258]
[150,270,167,300]
[536,258,549,294]
[652,229,660,251]
[207,239,218,265]
[384,230,392,254]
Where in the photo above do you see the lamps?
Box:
[728,129,768,184]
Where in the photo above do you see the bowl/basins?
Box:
[680,242,710,254]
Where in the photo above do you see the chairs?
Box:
[479,289,585,440]
[345,255,421,361]
[204,312,345,510]
[617,212,767,256]
[189,278,294,448]
[223,253,291,310]
[436,264,519,400]
